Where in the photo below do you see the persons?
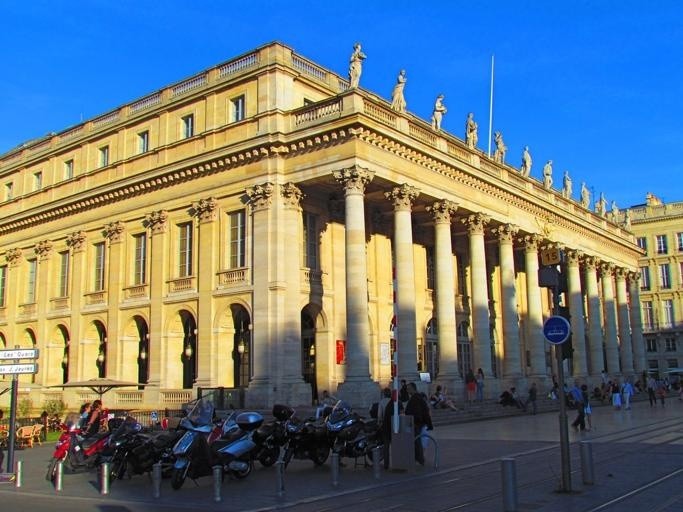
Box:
[40,401,107,439]
[0,409,5,472]
[321,390,338,407]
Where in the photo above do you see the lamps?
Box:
[236,303,245,359]
[95,332,104,366]
[61,329,68,368]
[139,321,147,365]
[183,317,192,361]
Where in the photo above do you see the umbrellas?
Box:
[56,377,151,410]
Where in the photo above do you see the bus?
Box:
[648,366,683,389]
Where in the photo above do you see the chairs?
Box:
[0,414,62,449]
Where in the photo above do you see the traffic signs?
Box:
[0,348,36,361]
[0,365,33,373]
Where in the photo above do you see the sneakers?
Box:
[570,422,579,432]
